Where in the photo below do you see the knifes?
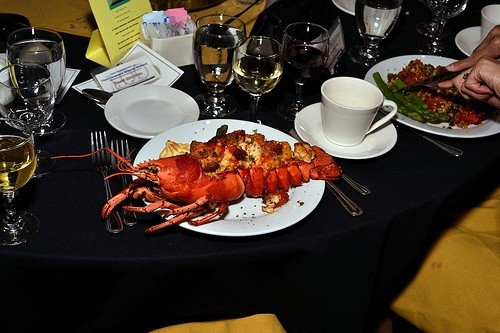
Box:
[81,87,113,103]
[397,53,500,93]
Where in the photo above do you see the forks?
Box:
[90,131,126,234]
[402,84,457,96]
[109,137,139,226]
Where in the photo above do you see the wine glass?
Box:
[349,0,403,66]
[191,13,247,118]
[416,0,468,38]
[232,34,284,127]
[5,26,67,135]
[0,117,40,247]
[281,20,330,121]
[0,63,55,178]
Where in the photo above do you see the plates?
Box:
[363,54,500,139]
[455,27,483,59]
[293,102,399,160]
[128,118,326,237]
[103,85,200,140]
[330,0,358,16]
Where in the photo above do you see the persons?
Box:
[436,25,500,108]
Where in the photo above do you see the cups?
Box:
[319,77,399,147]
[480,4,500,45]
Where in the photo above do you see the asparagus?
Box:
[373,72,452,124]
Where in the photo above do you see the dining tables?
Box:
[0,0,500,287]
